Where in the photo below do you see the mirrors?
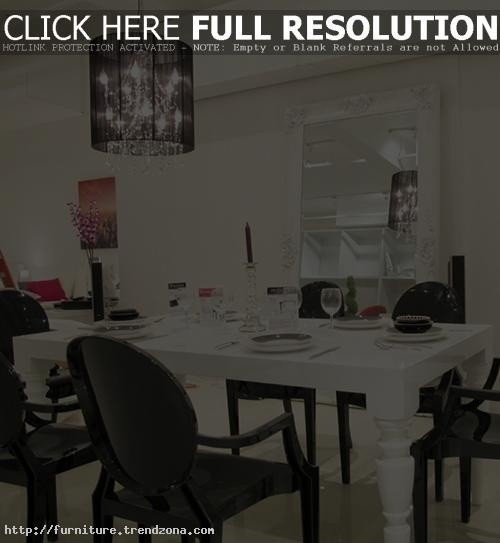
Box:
[279,82,441,279]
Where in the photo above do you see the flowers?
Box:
[66,200,106,262]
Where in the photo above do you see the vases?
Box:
[90,263,104,322]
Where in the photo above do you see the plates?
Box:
[89,306,452,354]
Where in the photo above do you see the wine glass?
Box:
[172,280,343,333]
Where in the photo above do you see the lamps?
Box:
[89,0,194,175]
[386,132,420,244]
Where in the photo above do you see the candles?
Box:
[245,222,253,263]
